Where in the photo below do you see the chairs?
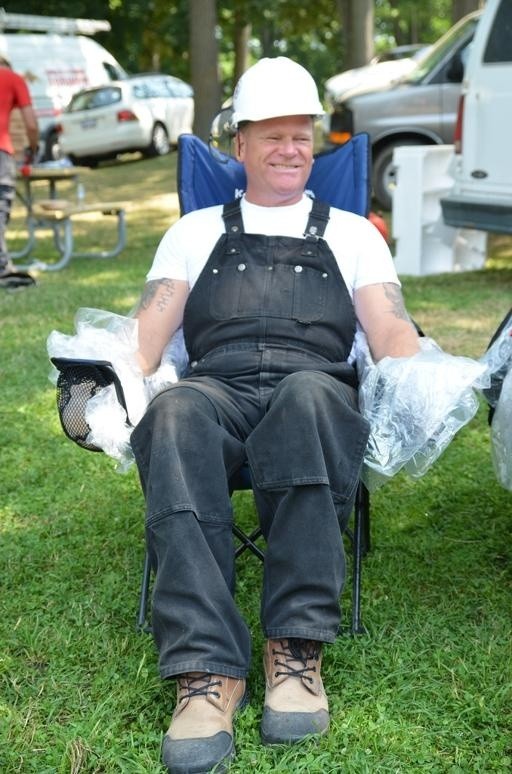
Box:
[139,132,371,632]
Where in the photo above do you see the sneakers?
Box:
[261,638,332,749]
[162,670,251,774]
[0,272,35,286]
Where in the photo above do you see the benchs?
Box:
[31,202,127,273]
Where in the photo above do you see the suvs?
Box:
[322,8,482,212]
[324,10,481,141]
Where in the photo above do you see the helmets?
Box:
[231,55,326,124]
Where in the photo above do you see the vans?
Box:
[439,0,510,236]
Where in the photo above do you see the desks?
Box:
[5,164,92,262]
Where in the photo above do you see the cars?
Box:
[58,72,196,169]
[371,42,432,63]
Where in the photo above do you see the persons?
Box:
[109,54,424,773]
[0,48,37,289]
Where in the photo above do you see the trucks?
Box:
[1,11,134,164]
